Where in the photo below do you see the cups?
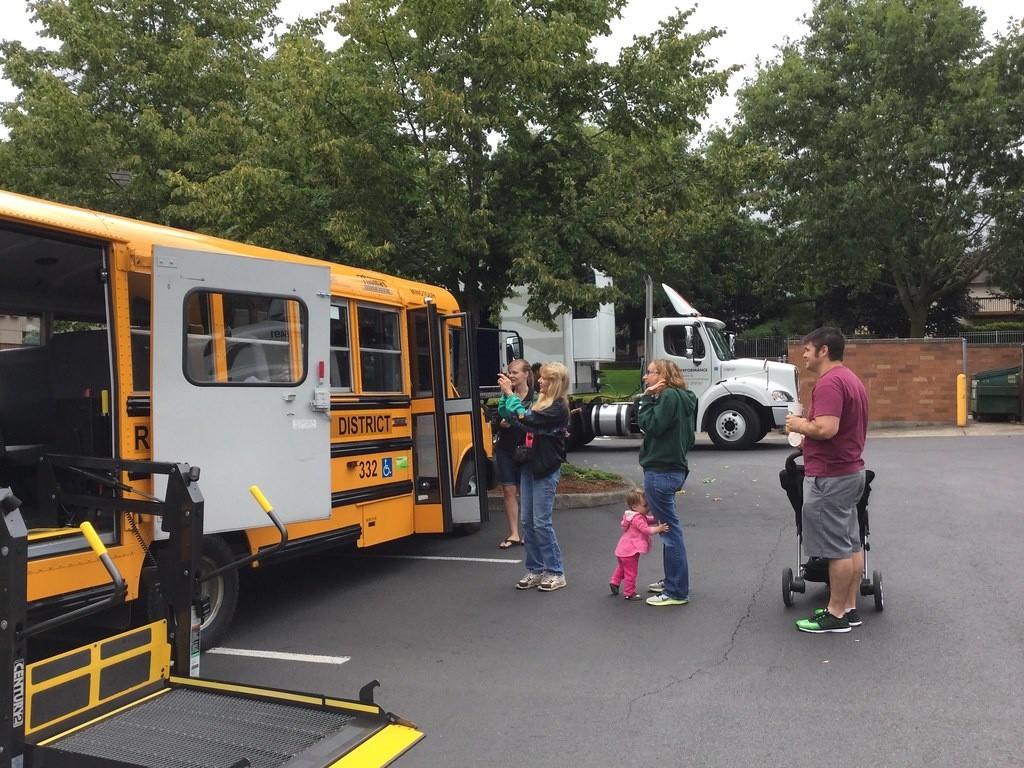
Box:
[788,432,805,447]
[788,403,802,418]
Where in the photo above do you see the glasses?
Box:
[646,370,663,375]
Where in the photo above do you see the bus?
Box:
[0,187,524,767]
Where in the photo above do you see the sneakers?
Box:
[813,608,862,625]
[626,592,642,601]
[610,584,619,595]
[516,572,543,589]
[796,607,851,633]
[646,593,689,606]
[648,579,665,592]
[537,570,567,591]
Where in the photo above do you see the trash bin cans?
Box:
[970,364,1023,424]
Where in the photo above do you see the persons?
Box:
[608,489,670,600]
[786,327,869,632]
[496,358,572,591]
[636,359,697,605]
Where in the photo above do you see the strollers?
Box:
[779,450,885,613]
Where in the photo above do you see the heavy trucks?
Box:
[437,263,803,452]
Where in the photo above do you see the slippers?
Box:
[499,538,521,549]
[521,542,525,545]
